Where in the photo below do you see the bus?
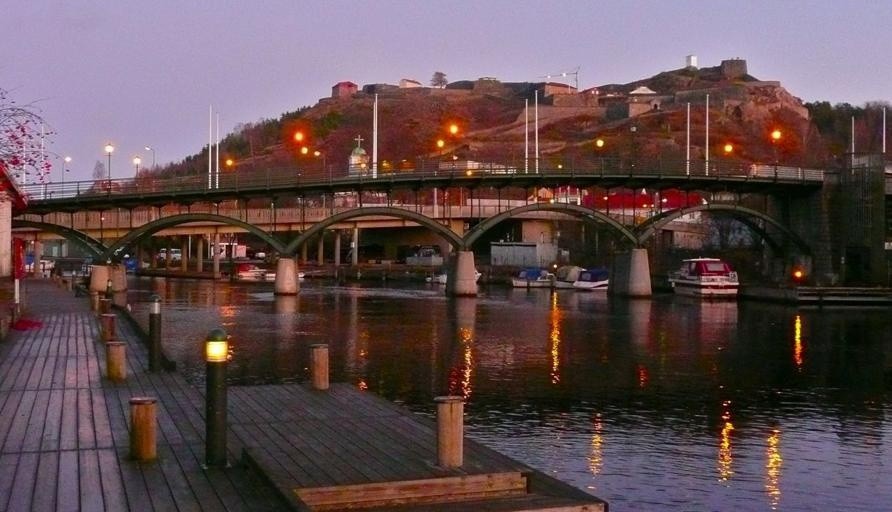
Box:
[406,244,442,266]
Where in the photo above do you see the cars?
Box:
[24,259,55,271]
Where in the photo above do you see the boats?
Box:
[667,255,740,298]
[232,264,305,281]
[425,266,483,284]
[511,264,610,293]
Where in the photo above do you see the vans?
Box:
[160,248,181,262]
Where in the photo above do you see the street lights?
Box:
[61,156,72,199]
[104,142,115,196]
[132,156,142,191]
[226,160,233,173]
[725,144,733,177]
[145,145,155,179]
[449,124,459,176]
[437,139,445,160]
[771,130,781,178]
[295,131,327,185]
[597,140,604,157]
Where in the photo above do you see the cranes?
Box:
[538,71,579,89]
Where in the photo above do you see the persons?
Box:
[49,264,59,283]
